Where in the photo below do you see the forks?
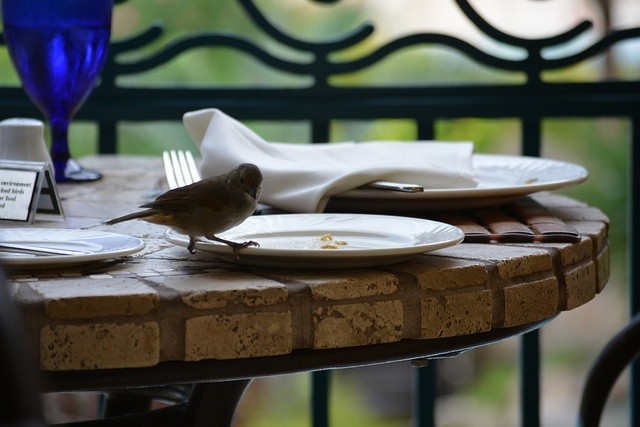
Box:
[162,149,201,189]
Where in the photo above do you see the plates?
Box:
[333,153,588,209]
[0,228,145,268]
[165,212,465,270]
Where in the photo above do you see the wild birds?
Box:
[108,162,264,266]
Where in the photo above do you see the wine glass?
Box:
[0,0,113,186]
